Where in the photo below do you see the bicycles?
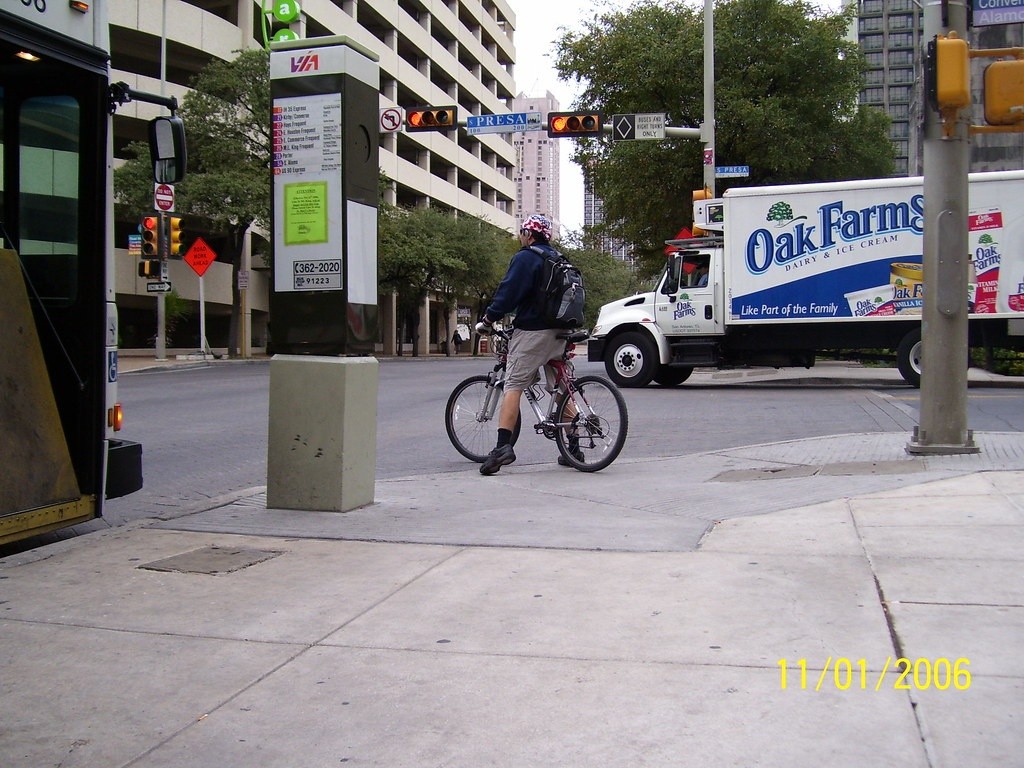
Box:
[446,325,629,474]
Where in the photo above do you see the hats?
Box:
[696,262,707,269]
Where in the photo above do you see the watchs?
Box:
[482,314,492,326]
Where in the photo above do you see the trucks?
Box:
[588,170,1024,385]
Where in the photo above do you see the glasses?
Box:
[520,229,532,235]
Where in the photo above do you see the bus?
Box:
[1,0,190,543]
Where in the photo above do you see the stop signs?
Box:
[153,181,175,212]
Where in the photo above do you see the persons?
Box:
[696,263,709,286]
[474,215,584,474]
[451,330,463,354]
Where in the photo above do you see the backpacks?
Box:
[519,245,586,328]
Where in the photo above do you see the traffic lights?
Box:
[166,215,186,258]
[692,185,711,236]
[138,215,158,256]
[924,35,973,116]
[549,112,601,138]
[404,106,457,133]
[140,261,158,277]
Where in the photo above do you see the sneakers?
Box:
[558,452,585,466]
[479,444,516,475]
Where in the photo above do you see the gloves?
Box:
[474,316,494,336]
